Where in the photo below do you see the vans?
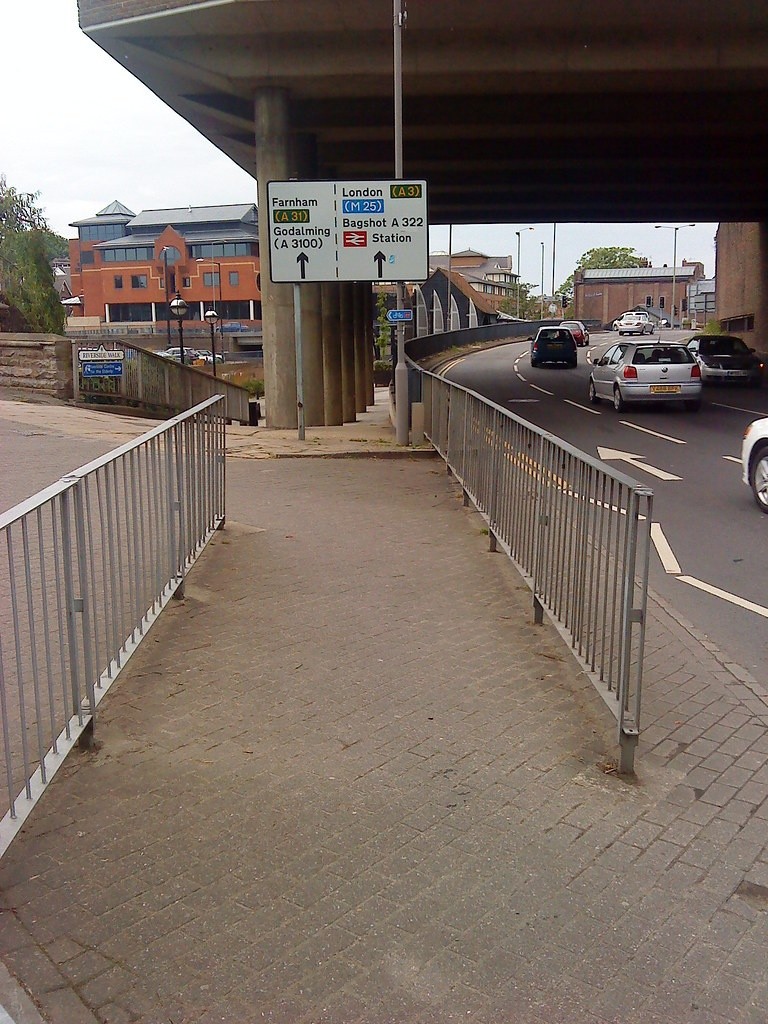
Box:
[612,311,649,331]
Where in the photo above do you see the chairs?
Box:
[635,353,646,363]
[541,333,549,338]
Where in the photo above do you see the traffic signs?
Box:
[265,179,430,281]
[82,361,123,377]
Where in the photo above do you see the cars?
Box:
[617,315,655,335]
[216,322,249,332]
[686,334,764,389]
[587,339,703,412]
[153,345,224,365]
[742,416,768,515]
[554,321,590,347]
[527,325,578,369]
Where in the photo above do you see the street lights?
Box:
[168,289,189,364]
[205,305,218,377]
[515,228,534,323]
[655,224,696,328]
